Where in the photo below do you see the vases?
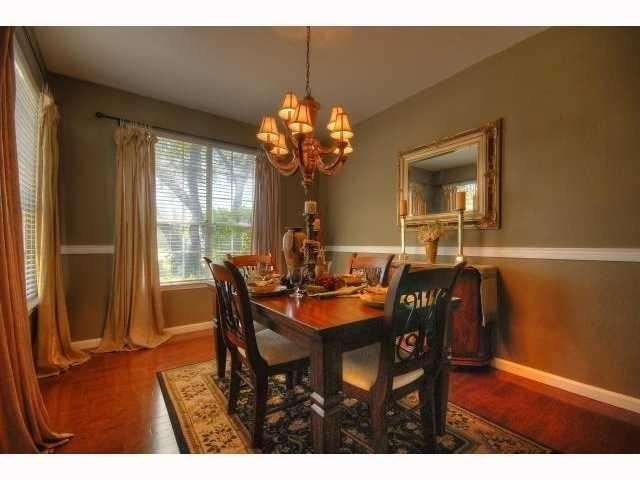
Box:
[283,227,307,289]
[424,237,438,264]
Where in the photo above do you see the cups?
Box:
[244,249,276,282]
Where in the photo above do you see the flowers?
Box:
[416,224,442,241]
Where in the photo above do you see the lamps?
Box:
[256,26,355,196]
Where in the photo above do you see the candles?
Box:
[313,219,320,231]
[305,201,317,214]
[400,200,408,215]
[456,192,466,209]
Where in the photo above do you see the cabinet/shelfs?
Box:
[388,262,498,366]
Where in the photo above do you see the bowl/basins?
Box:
[366,287,386,301]
[247,282,275,293]
[340,273,361,281]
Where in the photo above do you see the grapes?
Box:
[315,275,341,289]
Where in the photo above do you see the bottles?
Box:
[283,227,307,277]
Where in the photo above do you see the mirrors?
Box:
[393,118,503,231]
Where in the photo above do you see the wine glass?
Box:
[288,268,304,299]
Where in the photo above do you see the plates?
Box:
[244,286,286,298]
[360,294,416,309]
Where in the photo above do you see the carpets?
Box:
[155,351,567,454]
